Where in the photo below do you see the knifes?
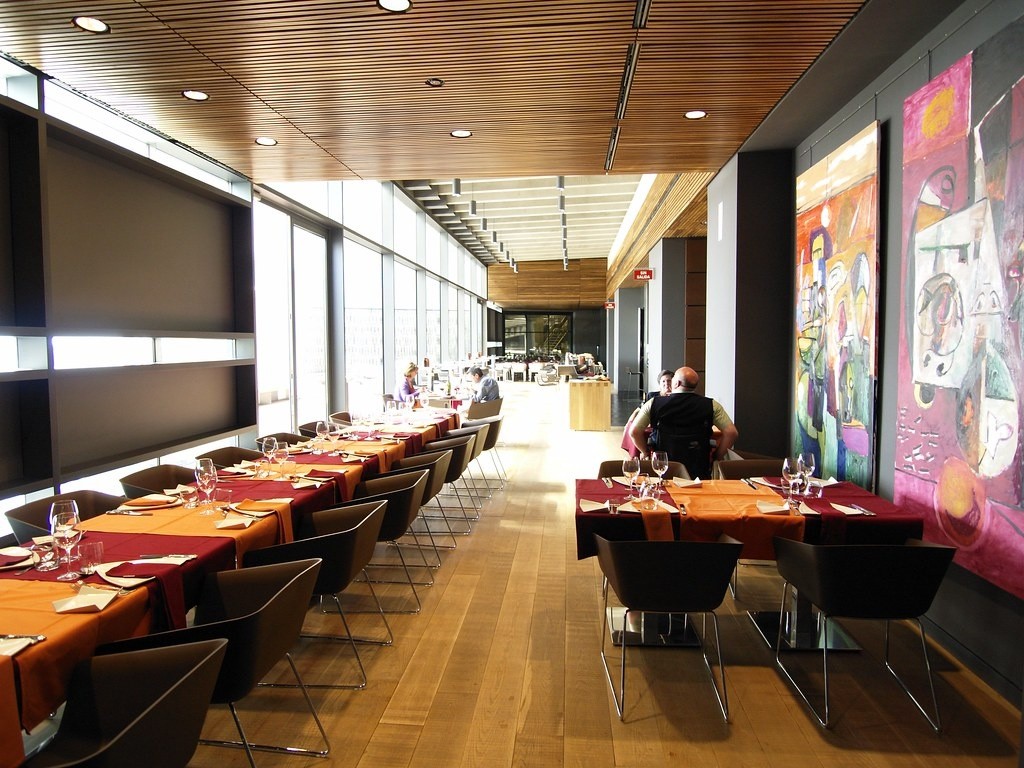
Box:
[851,504,876,516]
[106,510,153,516]
[680,504,686,516]
[741,478,756,491]
[140,554,198,558]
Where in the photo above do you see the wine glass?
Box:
[652,451,668,494]
[327,424,340,457]
[316,421,329,457]
[782,457,802,508]
[622,456,640,501]
[273,441,289,479]
[51,511,81,581]
[196,457,214,515]
[49,499,78,564]
[797,453,816,498]
[194,466,218,505]
[262,437,279,479]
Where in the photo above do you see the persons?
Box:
[470,368,499,403]
[628,366,738,481]
[525,352,533,368]
[398,362,420,404]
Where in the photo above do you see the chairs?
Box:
[0,394,505,768]
[593,460,957,736]
[494,362,544,382]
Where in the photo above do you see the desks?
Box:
[570,379,611,431]
[621,421,722,471]
[576,478,923,653]
[0,410,460,735]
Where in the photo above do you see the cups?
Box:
[77,542,104,575]
[29,541,60,571]
[398,402,406,414]
[282,460,296,481]
[420,393,429,408]
[213,488,232,511]
[405,394,415,407]
[255,459,270,478]
[387,401,396,414]
[636,473,651,496]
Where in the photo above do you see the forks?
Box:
[614,499,621,515]
[608,498,615,514]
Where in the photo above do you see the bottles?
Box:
[445,376,451,395]
[641,392,647,407]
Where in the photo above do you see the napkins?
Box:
[631,498,674,541]
[633,476,646,485]
[802,497,847,545]
[235,498,294,545]
[355,449,386,474]
[304,469,349,502]
[393,433,417,455]
[0,554,29,567]
[0,655,25,768]
[763,477,788,487]
[217,470,246,476]
[105,561,187,631]
[121,498,169,506]
